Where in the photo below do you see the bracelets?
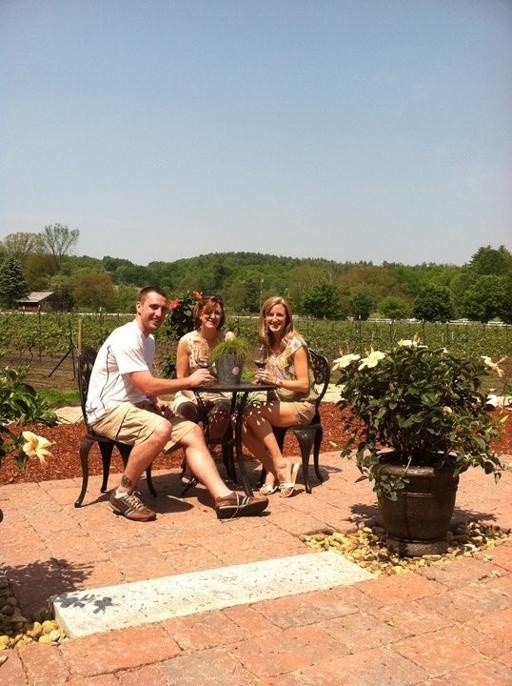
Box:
[274,376,282,384]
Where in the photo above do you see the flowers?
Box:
[0,363,65,474]
[331,334,511,470]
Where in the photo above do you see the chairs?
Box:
[257,348,330,494]
[160,360,234,481]
[74,346,158,508]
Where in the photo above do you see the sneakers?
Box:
[213,491,268,519]
[108,487,155,522]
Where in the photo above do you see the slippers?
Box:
[279,462,301,498]
[259,482,280,498]
[180,473,196,486]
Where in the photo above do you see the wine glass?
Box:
[198,348,212,386]
[194,350,198,364]
[254,348,268,386]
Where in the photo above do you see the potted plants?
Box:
[208,331,251,384]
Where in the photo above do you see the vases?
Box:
[368,451,459,541]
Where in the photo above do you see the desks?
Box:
[180,383,280,498]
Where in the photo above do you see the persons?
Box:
[172,295,234,484]
[242,297,319,497]
[85,287,269,522]
